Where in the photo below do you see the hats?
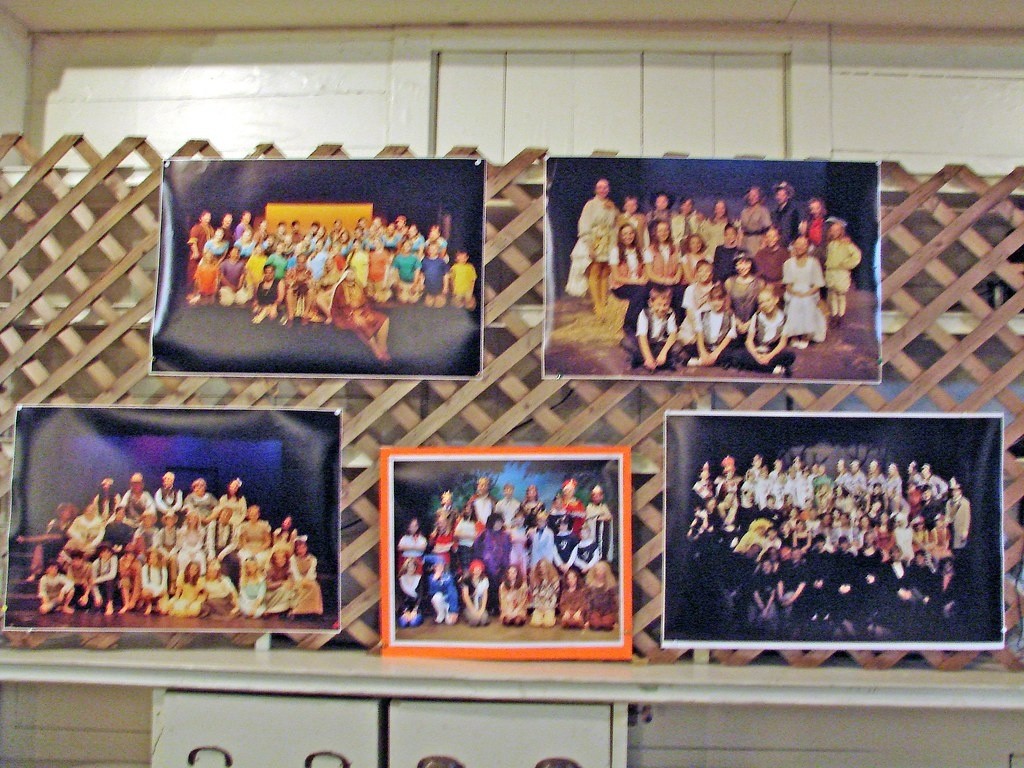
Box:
[162,511,178,523]
[96,542,114,554]
[141,510,157,526]
[562,479,576,494]
[826,217,848,227]
[469,560,485,575]
[772,181,795,198]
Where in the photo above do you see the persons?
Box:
[187,177,860,378]
[23,451,975,639]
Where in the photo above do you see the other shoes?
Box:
[791,335,799,347]
[683,356,701,367]
[795,337,810,350]
[827,311,845,329]
[632,356,642,369]
[780,365,793,380]
[665,359,677,369]
